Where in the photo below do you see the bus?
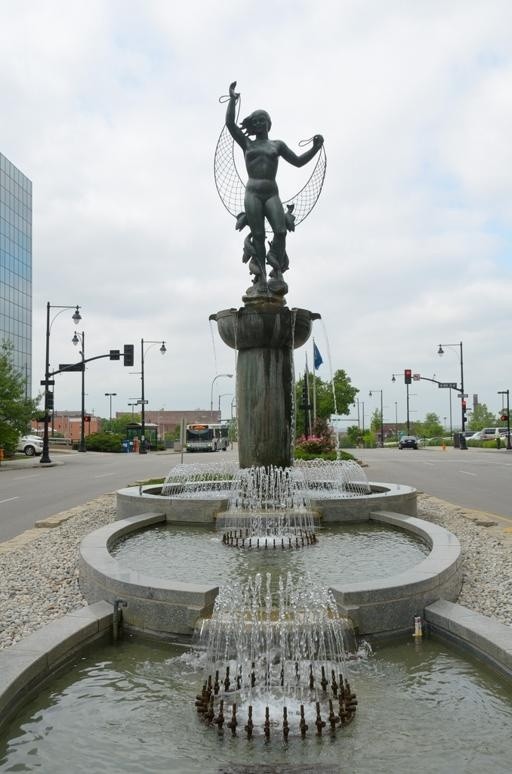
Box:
[185,424,229,452]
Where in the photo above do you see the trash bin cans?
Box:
[454,432,466,450]
[122,439,134,453]
[71,439,80,450]
[496,437,500,449]
[174,439,181,452]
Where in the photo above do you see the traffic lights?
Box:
[462,398,467,411]
[84,415,91,423]
[393,399,399,436]
[404,369,412,385]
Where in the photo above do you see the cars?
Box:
[416,427,511,449]
[398,435,418,450]
[14,434,43,457]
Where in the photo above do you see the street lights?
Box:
[210,373,234,423]
[37,301,83,464]
[139,338,167,455]
[437,341,465,433]
[368,389,384,448]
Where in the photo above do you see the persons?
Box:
[222,80,325,296]
